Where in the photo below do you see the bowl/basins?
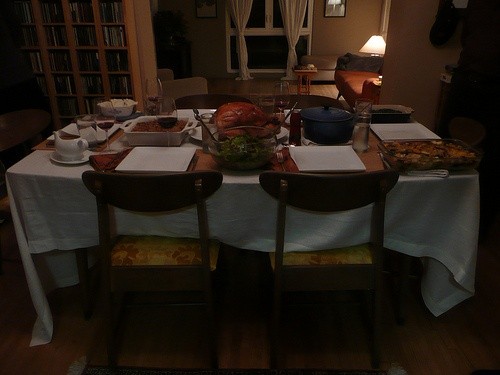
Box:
[119,115,198,146]
[207,126,277,170]
[377,137,484,172]
[97,99,139,117]
[371,105,415,122]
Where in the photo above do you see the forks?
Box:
[276,152,287,172]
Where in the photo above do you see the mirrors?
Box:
[323,0,347,18]
[195,0,218,18]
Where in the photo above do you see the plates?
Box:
[284,109,303,126]
[115,146,197,172]
[172,109,217,123]
[188,124,288,141]
[369,123,442,140]
[49,149,93,164]
[288,145,367,173]
[47,123,121,143]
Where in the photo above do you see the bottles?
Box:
[289,110,302,146]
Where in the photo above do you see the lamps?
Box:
[358,35,386,56]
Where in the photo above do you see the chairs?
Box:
[81,167,224,370]
[0,109,62,316]
[258,166,398,369]
[436,64,500,248]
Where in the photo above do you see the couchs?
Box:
[157,67,208,100]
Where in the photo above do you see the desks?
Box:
[293,68,319,96]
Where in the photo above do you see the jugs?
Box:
[53,129,88,161]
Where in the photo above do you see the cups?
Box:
[354,98,372,114]
[200,113,219,154]
[75,114,98,151]
[352,113,371,154]
[143,95,160,115]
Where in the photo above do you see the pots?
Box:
[300,105,354,145]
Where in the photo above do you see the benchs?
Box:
[332,53,383,106]
[300,54,337,81]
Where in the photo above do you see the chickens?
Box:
[214,102,282,141]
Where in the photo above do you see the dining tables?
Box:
[4,111,480,348]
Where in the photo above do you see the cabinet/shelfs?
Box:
[157,41,194,78]
[13,0,159,136]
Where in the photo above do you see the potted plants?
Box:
[153,10,188,49]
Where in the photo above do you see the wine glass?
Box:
[273,80,290,121]
[156,96,178,146]
[92,97,116,152]
[140,77,163,115]
